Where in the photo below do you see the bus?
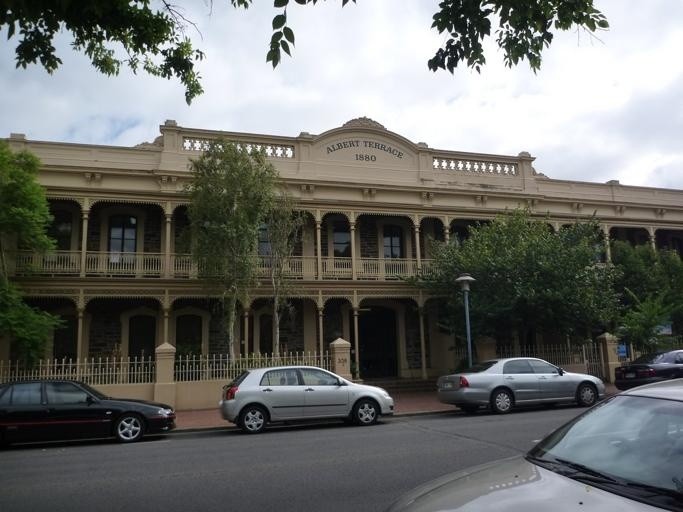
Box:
[436,357,605,414]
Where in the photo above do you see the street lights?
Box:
[457,274,476,368]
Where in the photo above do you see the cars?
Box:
[385,378,682,512]
[615,350,683,390]
[0,381,178,447]
[219,366,393,434]
